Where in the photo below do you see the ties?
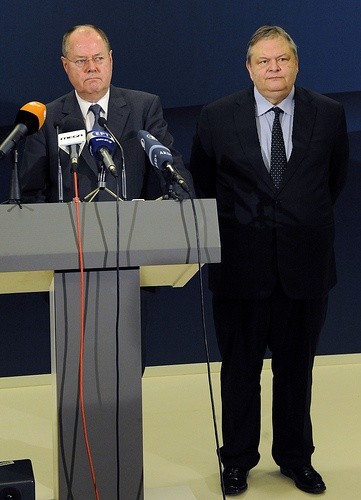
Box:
[89,105,105,132]
[270,107,288,189]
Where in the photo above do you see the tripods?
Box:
[80,160,124,202]
[156,175,184,201]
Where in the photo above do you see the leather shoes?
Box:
[280,465,327,492]
[222,468,249,495]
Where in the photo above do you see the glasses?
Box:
[63,54,109,66]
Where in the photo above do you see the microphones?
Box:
[53,117,127,203]
[138,130,190,193]
[0,101,47,161]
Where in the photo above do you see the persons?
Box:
[189,26,351,496]
[19,24,175,200]
